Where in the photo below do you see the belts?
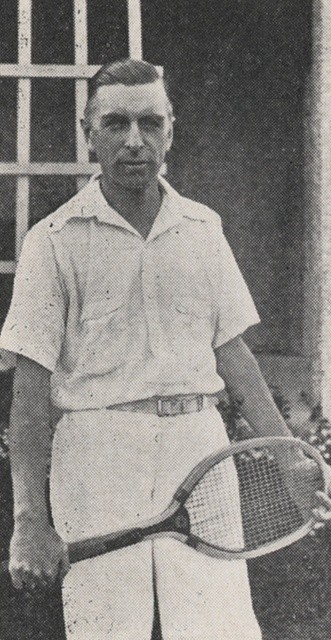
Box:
[106,394,219,417]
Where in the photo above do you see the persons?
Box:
[0,58,331,640]
